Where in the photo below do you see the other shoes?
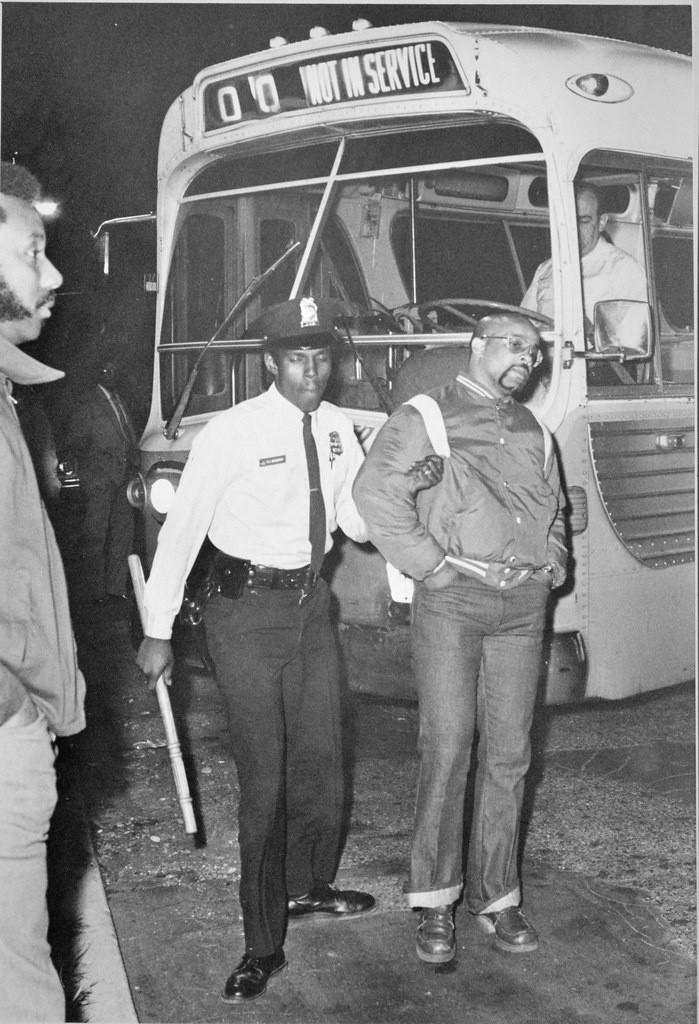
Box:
[95,599,108,608]
[108,593,128,601]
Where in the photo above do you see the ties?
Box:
[302,414,326,576]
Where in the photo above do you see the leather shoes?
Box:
[287,884,378,921]
[476,908,538,952]
[416,905,456,964]
[220,948,288,1004]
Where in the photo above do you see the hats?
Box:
[260,296,341,351]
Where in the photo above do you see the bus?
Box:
[90,16,699,712]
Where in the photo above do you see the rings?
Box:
[426,470,433,477]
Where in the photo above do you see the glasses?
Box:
[483,336,544,367]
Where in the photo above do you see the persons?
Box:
[519,180,654,387]
[352,312,573,964]
[135,295,446,1003]
[0,158,150,1024]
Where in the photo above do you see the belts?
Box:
[248,566,311,590]
